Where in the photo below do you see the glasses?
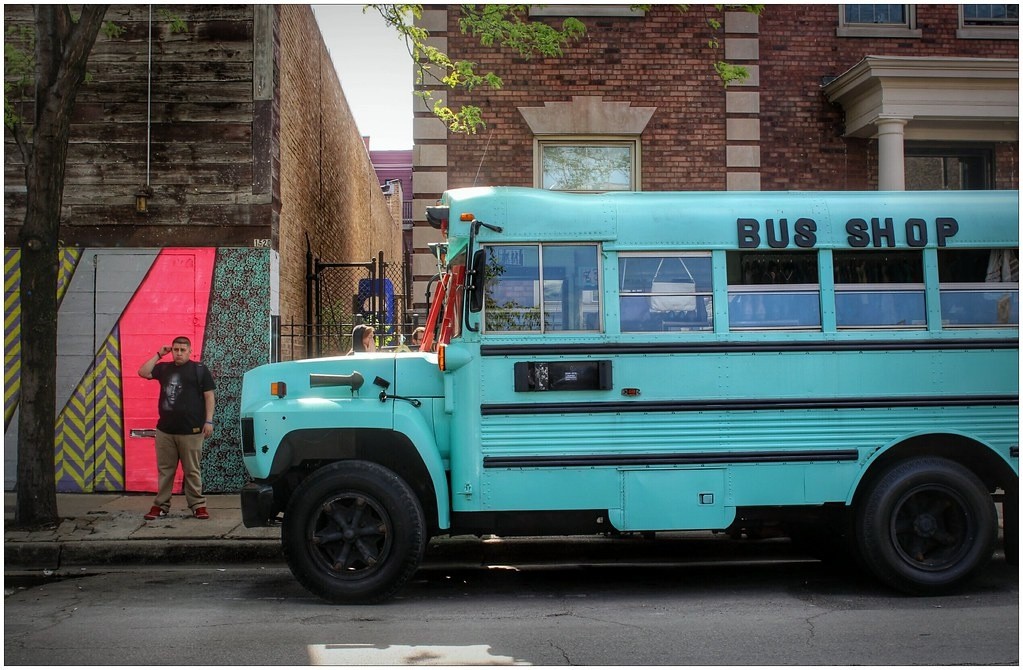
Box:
[415,339,423,341]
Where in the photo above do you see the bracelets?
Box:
[205,421,213,424]
[157,352,162,359]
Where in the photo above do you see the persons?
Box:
[138,336,217,519]
[347,325,378,355]
[412,327,432,352]
[389,335,401,346]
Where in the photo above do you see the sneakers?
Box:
[193,507,209,519]
[144,506,165,519]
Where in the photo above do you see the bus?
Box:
[239,185,1020,605]
